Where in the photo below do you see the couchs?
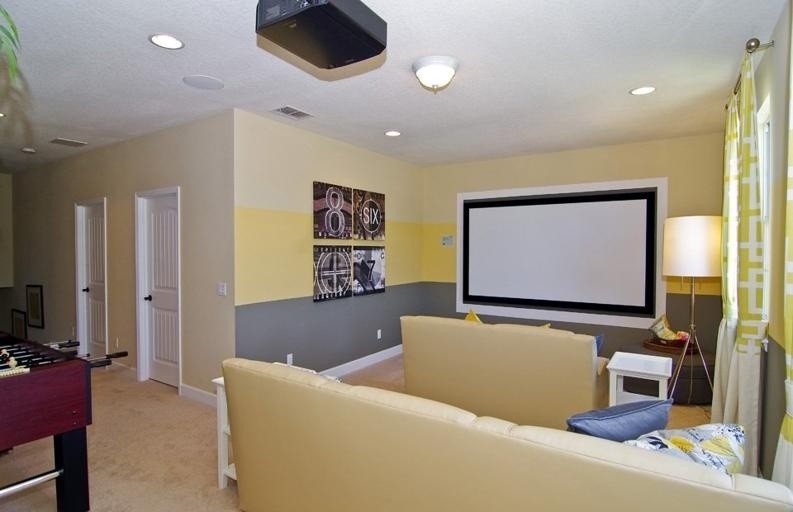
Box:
[220,357,792,512]
[399,315,608,430]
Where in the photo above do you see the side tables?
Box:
[211,377,238,489]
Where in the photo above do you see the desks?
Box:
[619,346,717,407]
[606,351,673,407]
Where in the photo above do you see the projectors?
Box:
[252,0,388,72]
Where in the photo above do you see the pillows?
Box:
[626,423,746,472]
[566,398,674,440]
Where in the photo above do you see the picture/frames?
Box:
[26,284,45,329]
[11,308,27,339]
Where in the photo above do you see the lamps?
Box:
[662,215,724,404]
[411,55,458,95]
